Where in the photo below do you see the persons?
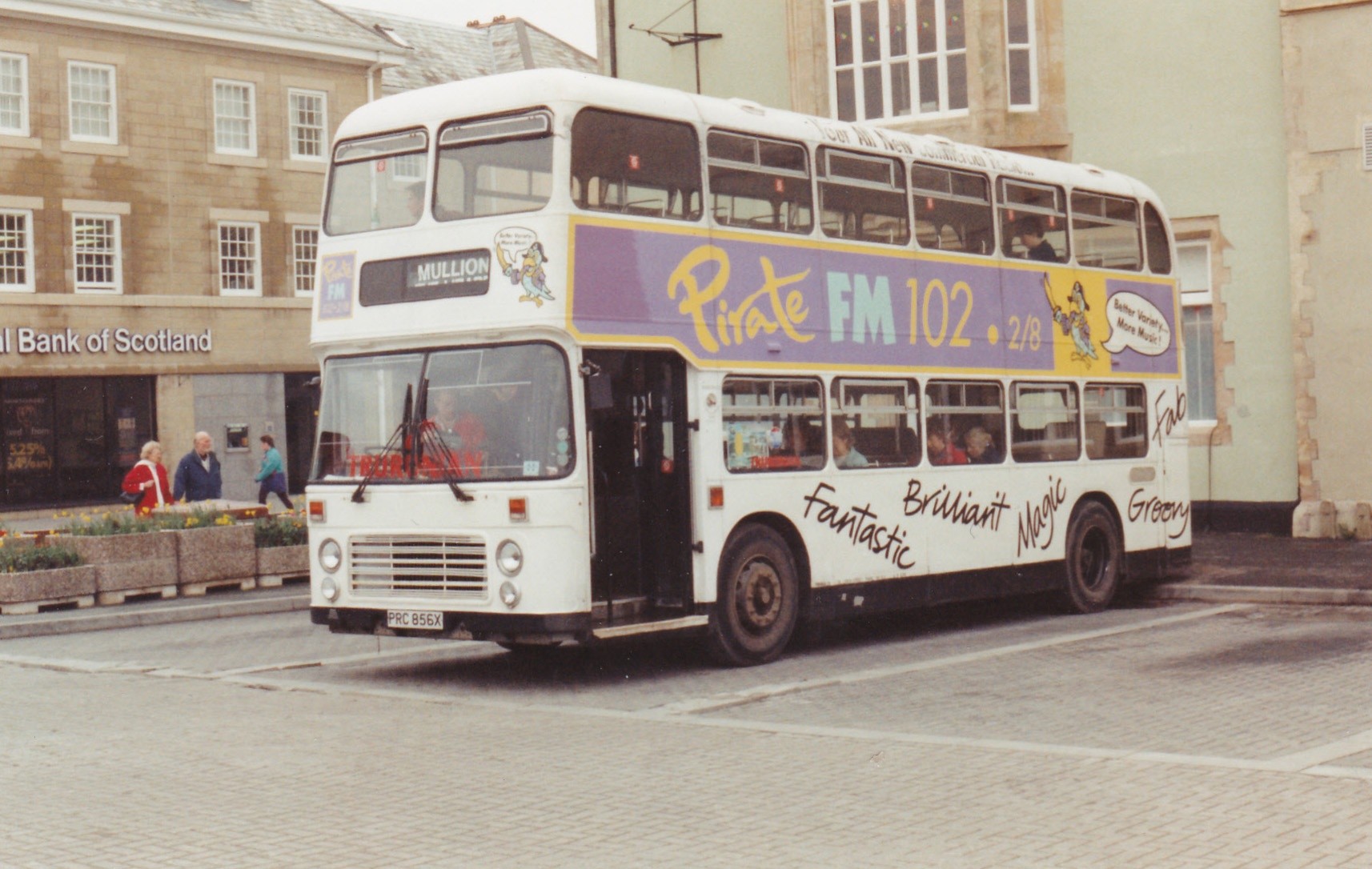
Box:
[253,434,294,509]
[781,415,1003,468]
[122,440,173,518]
[1015,216,1057,262]
[419,379,488,465]
[173,432,222,500]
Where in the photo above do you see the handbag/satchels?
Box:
[119,489,144,507]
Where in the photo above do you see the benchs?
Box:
[849,427,916,463]
[1006,426,1138,457]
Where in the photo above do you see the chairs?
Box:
[620,198,1137,271]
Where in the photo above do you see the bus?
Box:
[302,66,1191,666]
